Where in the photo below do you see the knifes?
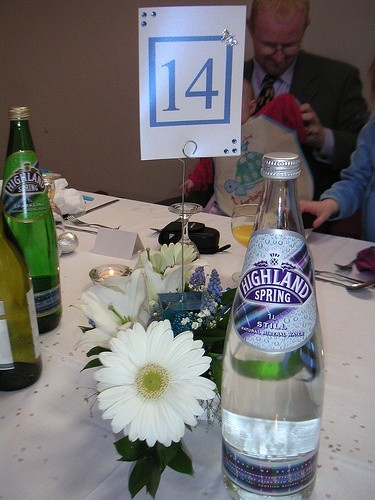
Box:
[64,224,97,234]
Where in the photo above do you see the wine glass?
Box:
[232,204,259,284]
[168,202,203,265]
[41,173,64,236]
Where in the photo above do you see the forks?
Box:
[66,215,121,230]
[334,258,357,271]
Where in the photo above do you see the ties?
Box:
[252,74,279,116]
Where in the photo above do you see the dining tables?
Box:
[0,177,375,500]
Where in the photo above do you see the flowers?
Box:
[67,240,240,500]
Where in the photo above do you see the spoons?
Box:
[315,276,375,291]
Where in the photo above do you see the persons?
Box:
[301,60,375,243]
[242,0,370,235]
[179,77,315,228]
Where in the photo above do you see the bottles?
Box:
[221,152,325,500]
[0,207,43,392]
[0,107,62,335]
